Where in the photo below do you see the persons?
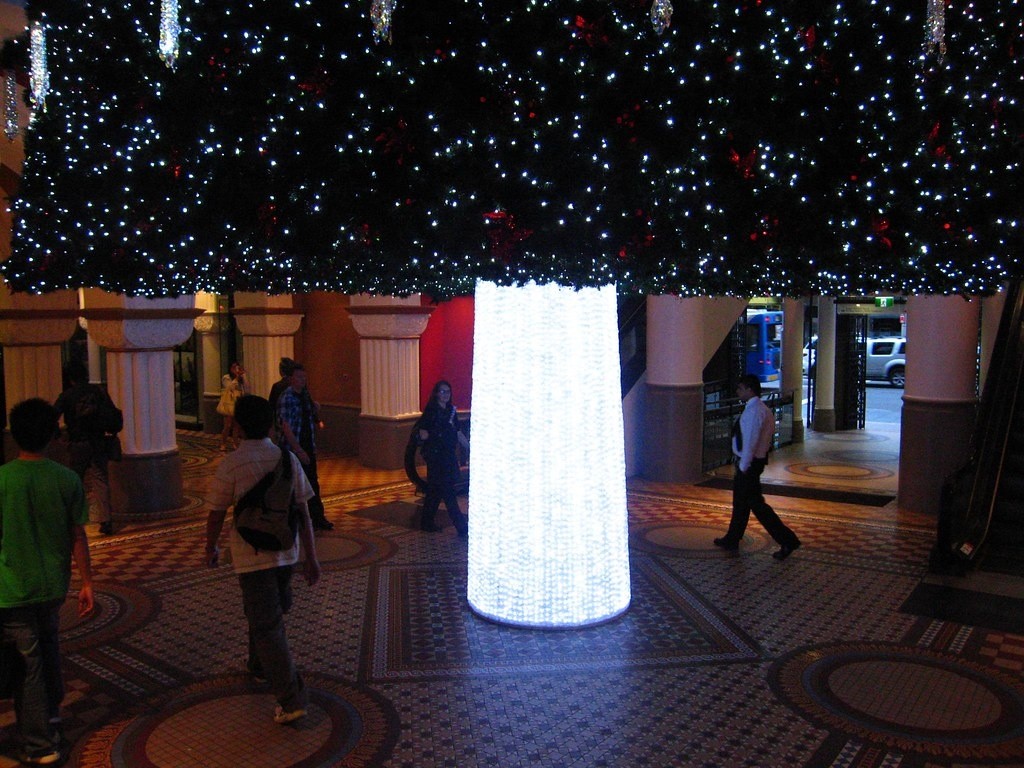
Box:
[0,397,96,764]
[714,374,802,561]
[216,360,251,451]
[269,357,334,534]
[202,393,321,723]
[51,360,123,537]
[418,380,470,537]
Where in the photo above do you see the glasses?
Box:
[436,390,452,396]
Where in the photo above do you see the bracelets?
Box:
[83,580,92,586]
[205,544,219,554]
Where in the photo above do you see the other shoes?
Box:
[274,699,308,723]
[248,671,270,687]
[219,444,237,451]
[774,537,802,561]
[714,537,739,551]
[98,523,113,534]
[420,522,441,532]
[458,525,468,538]
[312,518,333,531]
[14,743,60,764]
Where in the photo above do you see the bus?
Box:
[746,309,783,389]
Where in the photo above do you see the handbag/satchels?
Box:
[233,446,298,551]
[216,384,235,417]
[103,433,123,464]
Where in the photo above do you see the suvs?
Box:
[802,335,818,379]
[858,336,906,388]
